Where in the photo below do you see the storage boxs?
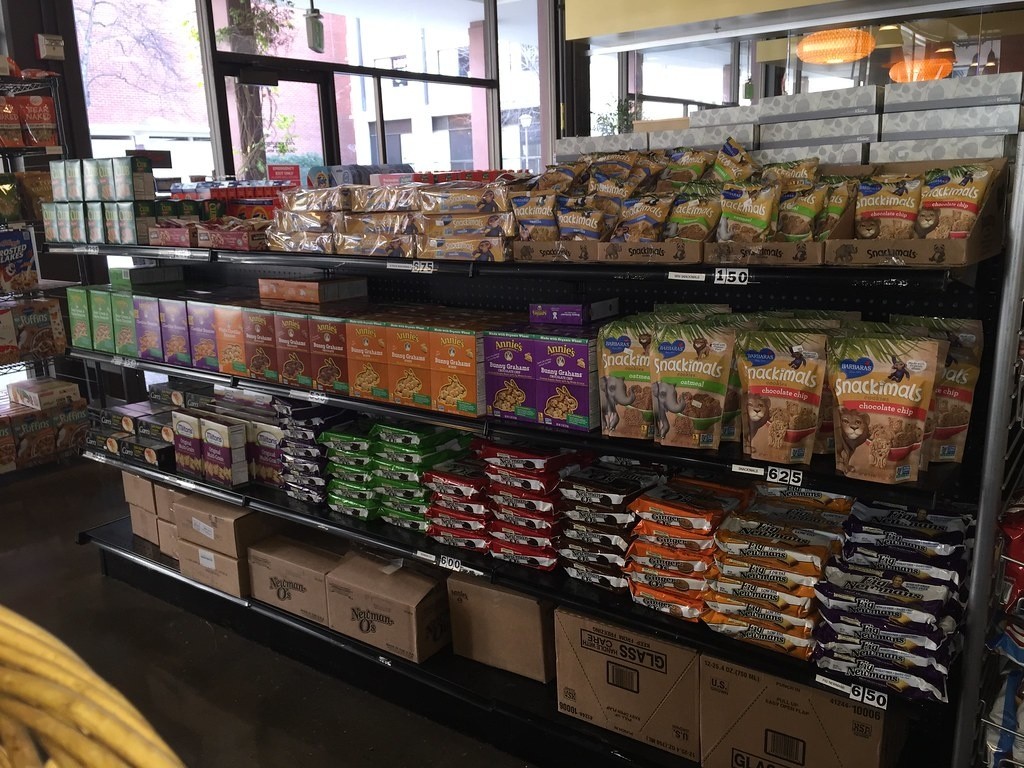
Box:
[760,114,882,149]
[0,156,1010,494]
[700,646,912,768]
[869,134,1018,164]
[0,155,1024,768]
[121,470,157,515]
[127,502,160,546]
[156,518,179,560]
[552,602,702,762]
[687,104,760,128]
[178,538,252,600]
[881,104,1024,142]
[174,494,296,559]
[153,483,191,522]
[757,84,885,126]
[649,123,759,154]
[326,546,451,666]
[555,131,649,162]
[244,525,351,629]
[447,570,554,683]
[751,142,868,167]
[883,71,1024,113]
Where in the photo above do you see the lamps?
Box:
[794,28,876,65]
[889,58,954,83]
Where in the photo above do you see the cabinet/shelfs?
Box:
[0,74,107,487]
[46,133,1024,768]
